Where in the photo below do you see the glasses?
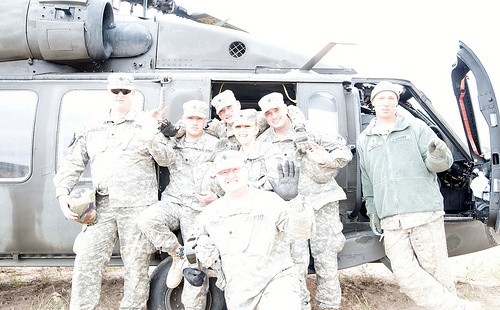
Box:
[111,89,132,95]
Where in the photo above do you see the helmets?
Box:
[67,188,97,224]
[192,235,223,279]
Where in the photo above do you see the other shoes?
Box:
[166,245,188,289]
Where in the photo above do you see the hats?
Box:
[258,92,284,115]
[210,90,236,114]
[233,109,257,128]
[106,72,134,90]
[370,81,399,101]
[182,99,209,119]
[213,150,243,175]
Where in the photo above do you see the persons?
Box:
[232,110,286,192]
[159,90,312,154]
[194,150,315,310]
[137,99,225,310]
[55,72,174,310]
[258,92,354,310]
[356,81,481,310]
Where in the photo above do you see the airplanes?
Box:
[0,2,500,310]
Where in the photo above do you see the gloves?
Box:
[428,139,448,157]
[268,160,300,201]
[156,119,181,139]
[293,124,312,155]
[182,268,206,287]
[369,214,383,236]
